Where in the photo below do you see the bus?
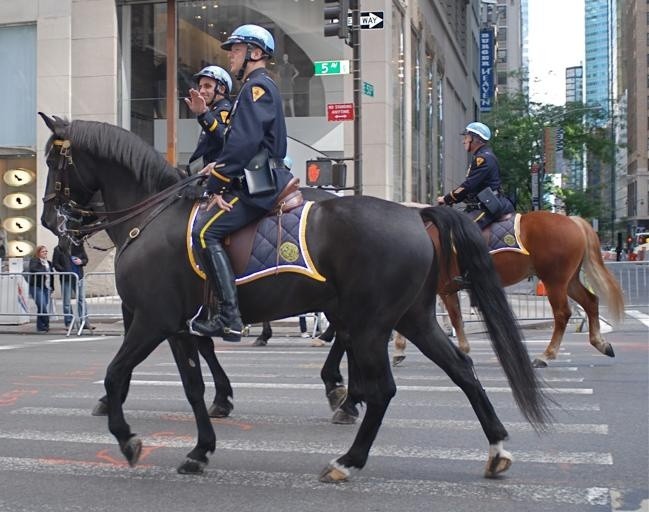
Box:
[633,233,649,254]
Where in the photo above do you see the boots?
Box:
[453,270,474,289]
[185,244,243,342]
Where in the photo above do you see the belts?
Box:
[268,158,285,169]
[467,194,500,209]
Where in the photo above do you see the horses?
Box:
[58,168,358,425]
[38,111,575,484]
[392,202,626,368]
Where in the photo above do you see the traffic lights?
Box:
[323,1,348,39]
[305,159,346,189]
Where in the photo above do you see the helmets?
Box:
[220,24,274,56]
[459,122,491,143]
[192,65,233,93]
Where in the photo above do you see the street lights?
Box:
[621,174,649,179]
[542,106,603,125]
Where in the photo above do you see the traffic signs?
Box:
[327,104,354,122]
[330,11,385,32]
[313,60,350,76]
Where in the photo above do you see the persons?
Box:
[437,122,506,284]
[195,24,293,341]
[29,245,54,332]
[53,243,96,331]
[184,65,233,167]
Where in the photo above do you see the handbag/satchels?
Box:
[243,148,277,195]
[476,186,503,215]
